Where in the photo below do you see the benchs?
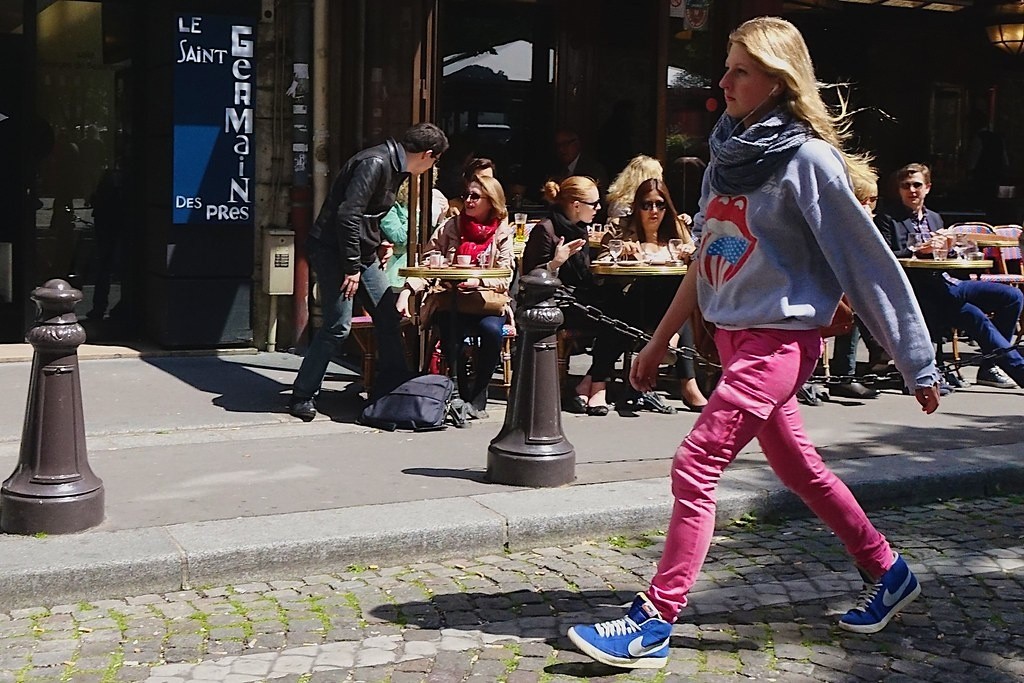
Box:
[349,315,516,400]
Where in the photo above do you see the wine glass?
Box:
[907,232,922,261]
[610,239,623,267]
[951,235,967,262]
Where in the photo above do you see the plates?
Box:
[428,265,449,268]
[592,260,614,266]
[451,264,476,268]
[644,259,670,264]
[617,261,645,265]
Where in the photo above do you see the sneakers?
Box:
[977,366,1016,387]
[838,555,923,633]
[567,590,672,669]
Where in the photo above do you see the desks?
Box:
[590,263,688,408]
[397,263,511,421]
[896,255,993,383]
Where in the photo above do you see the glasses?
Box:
[460,191,489,203]
[556,139,577,150]
[570,199,598,212]
[899,183,928,190]
[641,201,666,212]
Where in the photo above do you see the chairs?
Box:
[949,220,1024,342]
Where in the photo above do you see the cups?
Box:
[457,254,471,265]
[667,239,683,259]
[426,255,444,266]
[931,236,948,261]
[515,213,527,242]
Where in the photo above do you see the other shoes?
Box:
[831,377,880,397]
[447,365,466,398]
[86,306,102,319]
[287,393,315,416]
[682,395,706,412]
[588,398,607,415]
[567,387,589,412]
[469,385,486,414]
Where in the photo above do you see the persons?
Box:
[830,163,1024,396]
[566,15,941,668]
[85,168,128,318]
[293,124,708,419]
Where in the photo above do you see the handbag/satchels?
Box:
[456,287,512,318]
[361,370,453,430]
